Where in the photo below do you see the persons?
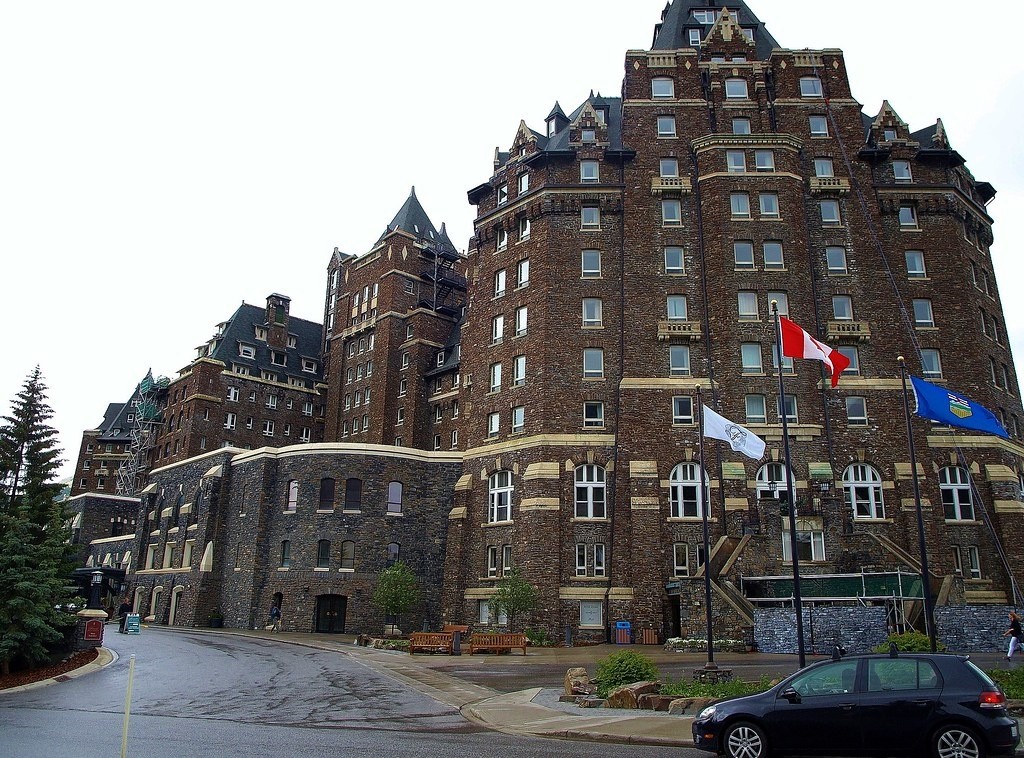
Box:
[118,598,130,633]
[1001,610,1024,662]
[270,603,281,634]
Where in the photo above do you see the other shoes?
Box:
[1003,656,1011,662]
[119,628,124,633]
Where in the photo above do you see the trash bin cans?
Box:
[615,621,631,644]
[642,622,658,645]
[75,608,108,651]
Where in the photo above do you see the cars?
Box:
[691,640,1021,758]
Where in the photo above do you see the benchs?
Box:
[470,633,527,656]
[409,631,454,656]
[437,624,470,639]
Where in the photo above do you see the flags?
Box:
[908,373,1008,439]
[702,405,766,461]
[778,313,851,388]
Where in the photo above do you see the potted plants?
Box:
[207,613,225,627]
